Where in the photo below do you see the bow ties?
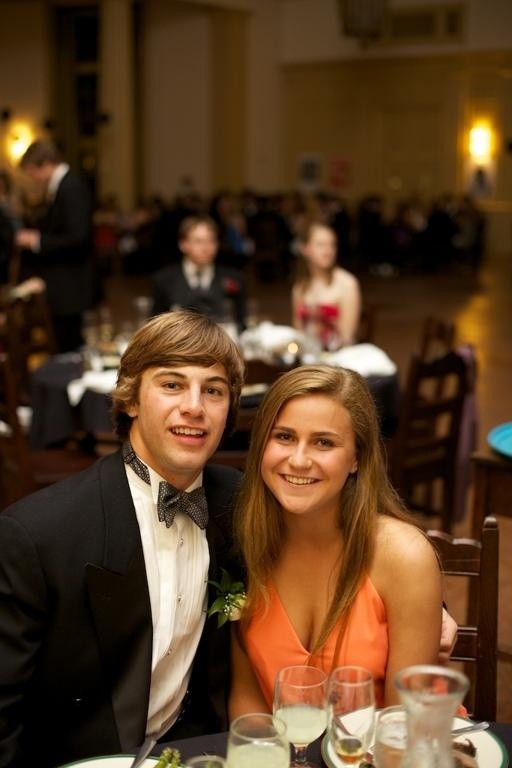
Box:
[157,481,210,530]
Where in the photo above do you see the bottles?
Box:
[392,664,471,768]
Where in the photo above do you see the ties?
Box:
[197,269,204,291]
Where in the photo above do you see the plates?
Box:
[321,706,509,768]
[57,753,189,767]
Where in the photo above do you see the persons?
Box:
[1,141,493,350]
[229,364,444,730]
[0,309,244,768]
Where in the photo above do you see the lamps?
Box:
[466,115,499,188]
[1,105,41,166]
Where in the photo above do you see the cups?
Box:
[185,755,226,768]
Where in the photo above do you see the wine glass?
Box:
[376,705,408,768]
[226,712,292,768]
[272,665,329,768]
[329,666,377,768]
